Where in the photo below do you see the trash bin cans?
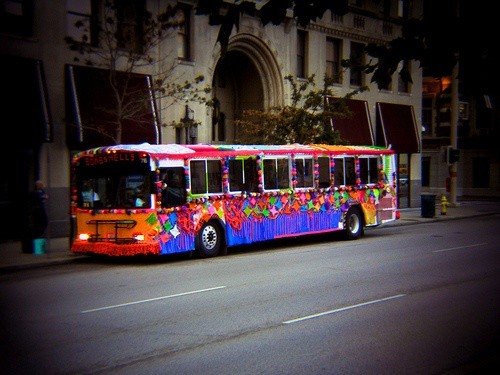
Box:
[33,238,49,256]
[420,194,437,218]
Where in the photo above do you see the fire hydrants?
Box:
[441,194,448,215]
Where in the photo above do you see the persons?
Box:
[30,180,49,240]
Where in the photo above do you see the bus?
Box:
[70,143,399,259]
